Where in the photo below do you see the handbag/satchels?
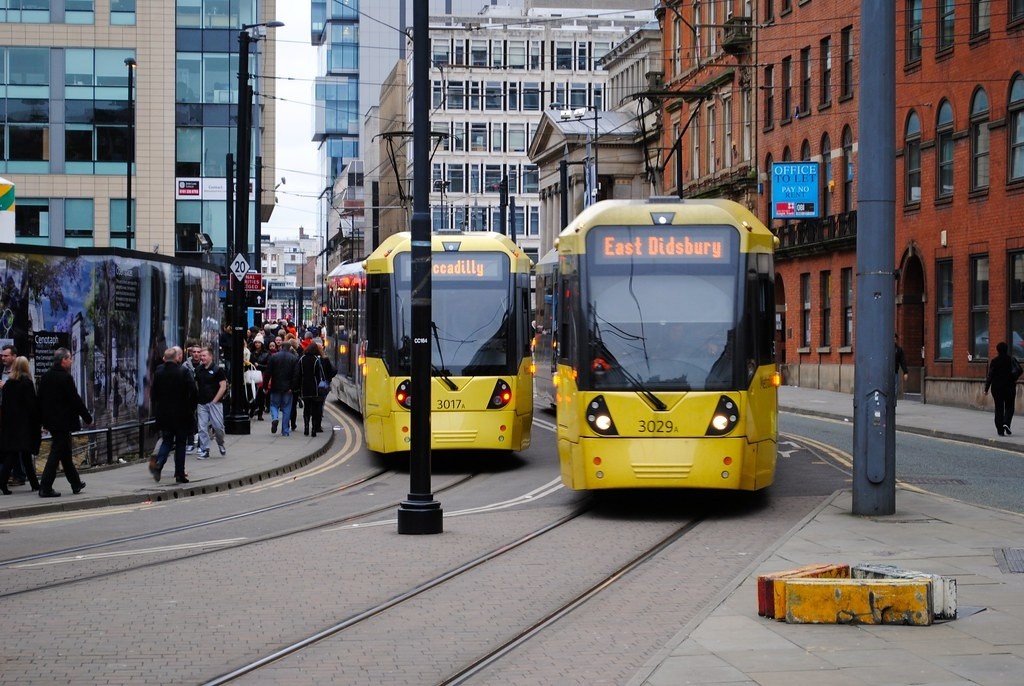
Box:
[243,364,262,384]
[318,378,330,393]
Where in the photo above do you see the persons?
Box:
[95,358,153,424]
[0,354,41,496]
[184,337,202,357]
[296,341,331,438]
[34,347,95,498]
[208,311,339,433]
[193,346,228,460]
[148,345,185,473]
[646,321,726,367]
[150,348,190,484]
[983,341,1024,437]
[180,343,204,455]
[894,333,909,415]
[0,343,17,390]
[262,340,300,437]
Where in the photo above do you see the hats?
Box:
[253,332,265,344]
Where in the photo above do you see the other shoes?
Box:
[185,445,201,455]
[172,472,189,478]
[0,484,12,495]
[9,479,26,486]
[250,407,323,437]
[149,454,157,476]
[38,487,62,497]
[154,463,161,483]
[219,445,226,456]
[1002,424,1012,435]
[31,481,40,491]
[176,476,190,483]
[196,451,210,460]
[73,480,86,494]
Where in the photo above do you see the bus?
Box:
[536,195,787,492]
[326,228,538,454]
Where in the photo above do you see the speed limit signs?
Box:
[229,253,249,282]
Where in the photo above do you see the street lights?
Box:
[223,22,291,435]
[121,58,137,250]
[549,101,598,206]
